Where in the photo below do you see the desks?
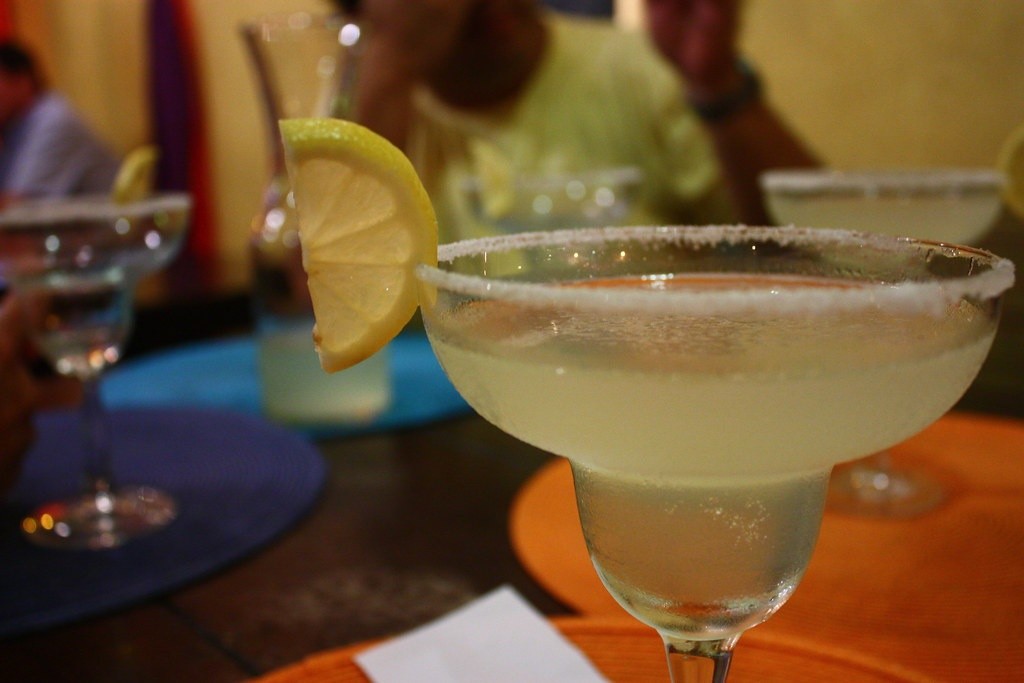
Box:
[0,222,1024,683]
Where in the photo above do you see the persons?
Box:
[287,0,820,330]
[0,38,123,429]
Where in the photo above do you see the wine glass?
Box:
[0,190,193,548]
[756,171,1008,517]
[418,224,1015,683]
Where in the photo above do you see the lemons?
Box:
[278,119,437,371]
[1000,126,1024,218]
[110,145,155,212]
[475,141,513,218]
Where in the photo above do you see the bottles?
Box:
[240,15,397,427]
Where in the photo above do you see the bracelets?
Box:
[696,56,764,122]
